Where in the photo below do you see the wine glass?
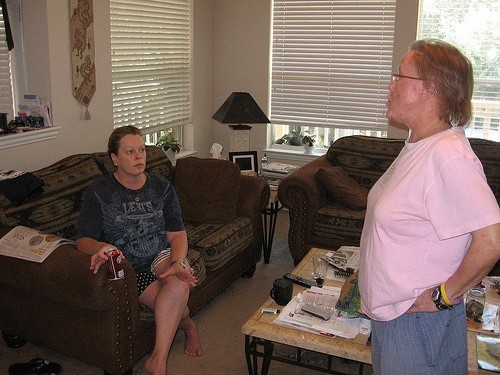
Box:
[312,253,329,289]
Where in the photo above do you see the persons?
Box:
[359,37,500,375]
[74,125,202,375]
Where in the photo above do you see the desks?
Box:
[241,247,500,375]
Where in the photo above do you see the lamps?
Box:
[211,91,271,130]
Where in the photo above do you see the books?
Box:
[17,93,53,128]
[0,226,77,263]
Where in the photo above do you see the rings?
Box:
[190,268,194,274]
[179,261,185,268]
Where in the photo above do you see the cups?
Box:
[269,278,293,306]
[15,117,44,128]
[0,113,7,130]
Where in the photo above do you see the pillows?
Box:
[278,134,500,275]
[315,166,367,209]
[175,157,240,223]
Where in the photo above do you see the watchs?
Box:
[431,284,450,310]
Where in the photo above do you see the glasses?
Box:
[391,74,438,95]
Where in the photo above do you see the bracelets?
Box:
[440,282,453,306]
[171,259,186,265]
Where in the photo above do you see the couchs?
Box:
[0,143,270,375]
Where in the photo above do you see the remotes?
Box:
[320,256,349,271]
[301,304,331,321]
[283,273,318,288]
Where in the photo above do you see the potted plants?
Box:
[156,129,181,159]
[302,129,318,155]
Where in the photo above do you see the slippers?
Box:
[3,332,27,348]
[9,357,63,375]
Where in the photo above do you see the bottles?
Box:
[465,287,486,321]
[262,154,267,167]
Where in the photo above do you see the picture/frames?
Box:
[228,150,258,177]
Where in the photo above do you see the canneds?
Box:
[103,248,125,280]
